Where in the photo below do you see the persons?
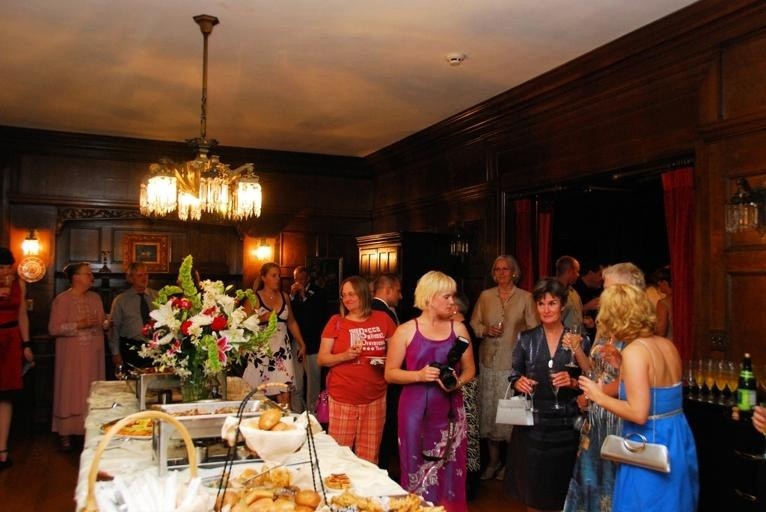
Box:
[314,274,396,464]
[371,272,402,325]
[0,248,32,466]
[237,261,306,412]
[288,265,321,412]
[48,260,112,451]
[732,402,765,434]
[110,259,166,377]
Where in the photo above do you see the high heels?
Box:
[478,458,501,480]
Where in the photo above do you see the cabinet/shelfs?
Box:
[353,228,438,314]
[679,385,766,512]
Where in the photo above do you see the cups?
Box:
[354,338,363,351]
[755,365,766,390]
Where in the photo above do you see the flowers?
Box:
[123,249,277,405]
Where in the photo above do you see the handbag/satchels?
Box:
[313,388,331,425]
[490,377,537,429]
[596,431,675,476]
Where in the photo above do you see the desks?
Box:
[76,375,440,512]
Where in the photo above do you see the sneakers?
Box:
[58,437,73,452]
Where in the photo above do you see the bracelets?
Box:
[22,340,30,352]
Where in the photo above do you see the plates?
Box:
[100,416,155,440]
[17,256,47,282]
[364,356,387,359]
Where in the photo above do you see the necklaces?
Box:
[263,293,276,300]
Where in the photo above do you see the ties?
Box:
[136,292,152,327]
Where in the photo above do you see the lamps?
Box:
[136,12,263,221]
[718,174,765,239]
[450,218,477,264]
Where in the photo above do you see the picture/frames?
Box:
[120,231,172,275]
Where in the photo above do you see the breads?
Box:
[247,408,297,432]
[216,464,321,512]
[325,473,352,489]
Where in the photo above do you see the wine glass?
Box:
[491,321,503,345]
[685,358,737,407]
[564,322,581,369]
[115,364,126,384]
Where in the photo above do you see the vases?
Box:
[178,366,212,405]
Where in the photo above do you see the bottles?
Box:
[190,440,210,464]
[99,251,111,273]
[735,352,757,420]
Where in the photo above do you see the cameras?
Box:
[429,336,470,388]
